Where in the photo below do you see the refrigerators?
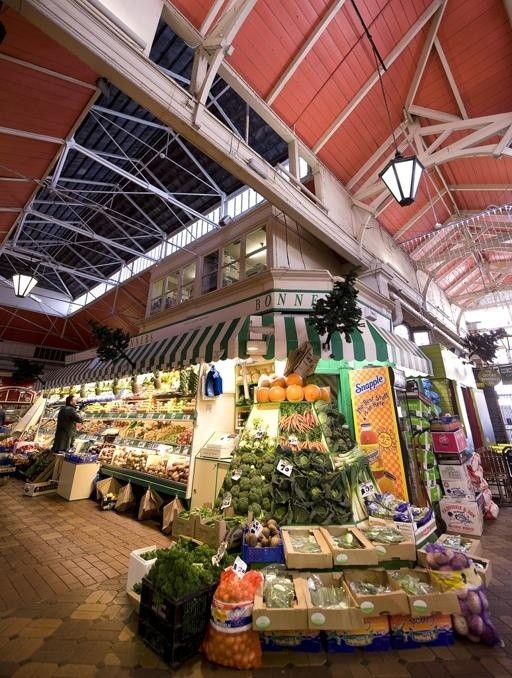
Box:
[339,367,427,507]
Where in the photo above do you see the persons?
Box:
[53,394,87,451]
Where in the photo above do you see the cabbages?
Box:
[274,444,353,523]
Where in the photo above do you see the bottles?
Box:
[360,422,384,478]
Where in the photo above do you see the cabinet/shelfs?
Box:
[234,361,287,430]
[19,405,194,498]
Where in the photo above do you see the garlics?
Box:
[426,547,497,644]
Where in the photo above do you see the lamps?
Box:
[372,48,423,207]
[12,263,38,297]
[424,177,442,229]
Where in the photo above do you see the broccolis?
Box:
[216,452,275,518]
